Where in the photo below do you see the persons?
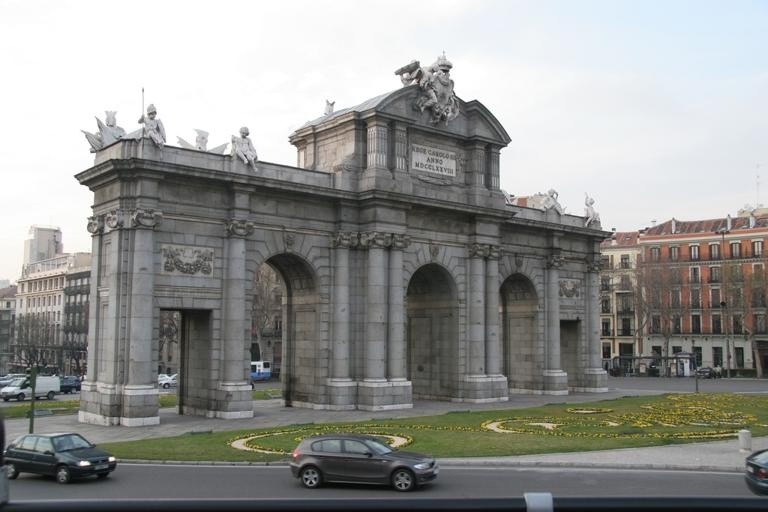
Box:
[231,126,258,172]
[584,197,599,228]
[138,104,166,150]
[417,64,442,112]
[539,188,567,216]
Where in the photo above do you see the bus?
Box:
[251,360,272,382]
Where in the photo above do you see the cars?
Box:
[696,367,715,378]
[1,431,117,484]
[745,447,768,496]
[159,374,178,389]
[0,373,86,402]
[159,373,170,381]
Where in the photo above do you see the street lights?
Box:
[721,300,731,377]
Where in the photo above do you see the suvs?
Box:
[290,434,441,491]
